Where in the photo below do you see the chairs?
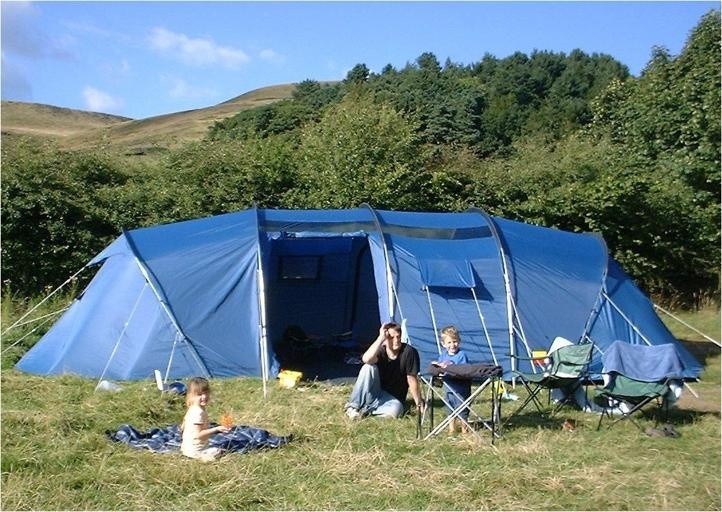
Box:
[504,342,593,426]
[594,340,684,433]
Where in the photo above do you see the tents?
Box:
[14,202,703,382]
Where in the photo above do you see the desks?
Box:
[417,372,502,446]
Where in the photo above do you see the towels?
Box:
[601,339,682,382]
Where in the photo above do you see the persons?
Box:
[180,376,231,463]
[344,322,425,421]
[438,324,471,437]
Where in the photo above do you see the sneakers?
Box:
[347,407,360,421]
